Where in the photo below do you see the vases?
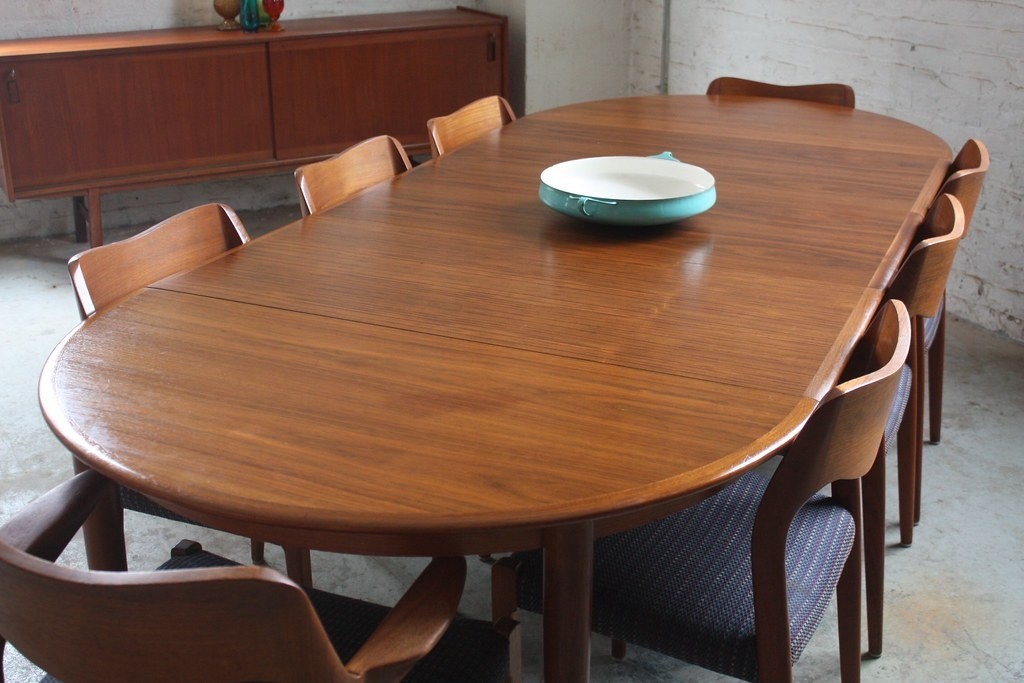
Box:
[214,0,286,33]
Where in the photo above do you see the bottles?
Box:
[240,0,259,30]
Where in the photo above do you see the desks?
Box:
[32,89,955,683]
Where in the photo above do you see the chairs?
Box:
[291,134,414,217]
[494,296,910,683]
[917,137,989,446]
[68,201,312,591]
[424,96,520,163]
[0,471,522,683]
[775,194,964,662]
[705,73,859,109]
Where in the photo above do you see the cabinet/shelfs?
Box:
[0,5,510,244]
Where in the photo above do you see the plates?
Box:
[540,150,717,225]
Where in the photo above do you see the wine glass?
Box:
[262,0,285,32]
[213,0,241,31]
